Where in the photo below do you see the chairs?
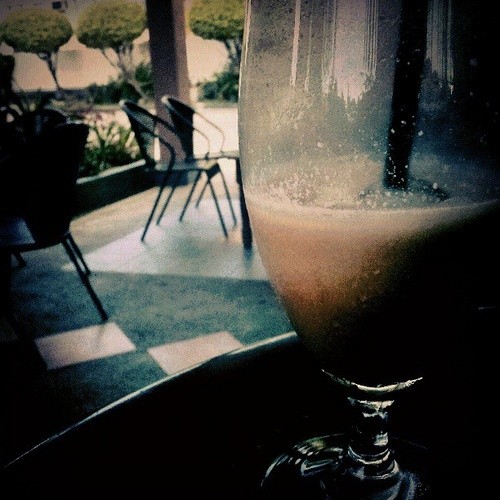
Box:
[1,106,109,371]
[119,96,241,241]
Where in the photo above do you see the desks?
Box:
[0,329,500,500]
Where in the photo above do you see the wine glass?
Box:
[237,0,500,500]
